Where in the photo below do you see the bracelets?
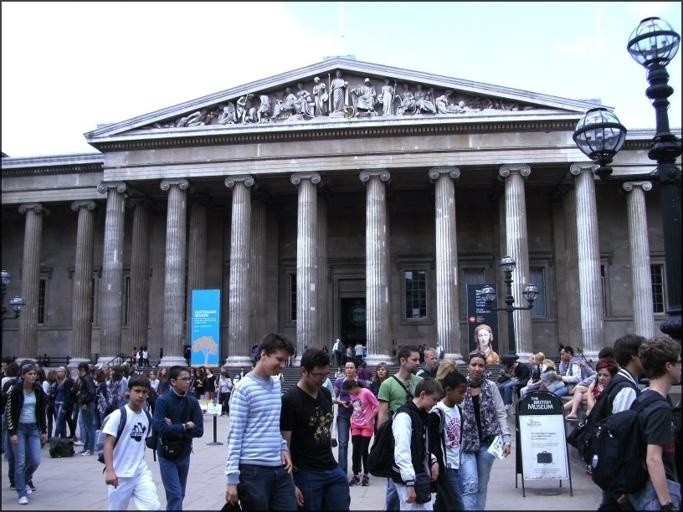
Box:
[505,443,511,446]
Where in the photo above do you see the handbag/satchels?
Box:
[50,438,74,458]
[415,474,431,503]
[159,439,186,460]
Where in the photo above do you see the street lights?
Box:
[481,254,541,371]
[0,269,26,364]
[571,13,683,343]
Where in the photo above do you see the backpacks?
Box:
[567,379,667,494]
[366,406,414,476]
[95,407,126,464]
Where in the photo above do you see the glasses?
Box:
[309,369,330,379]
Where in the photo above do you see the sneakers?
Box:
[11,480,35,504]
[349,475,360,485]
[362,476,369,486]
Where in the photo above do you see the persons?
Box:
[597,335,646,510]
[322,338,366,368]
[102,375,161,510]
[154,366,203,511]
[378,346,424,511]
[323,361,391,487]
[5,364,48,504]
[417,347,440,380]
[1,345,246,491]
[629,334,682,511]
[467,325,500,364]
[498,345,613,419]
[429,353,511,511]
[391,378,444,511]
[281,348,351,509]
[224,332,298,511]
